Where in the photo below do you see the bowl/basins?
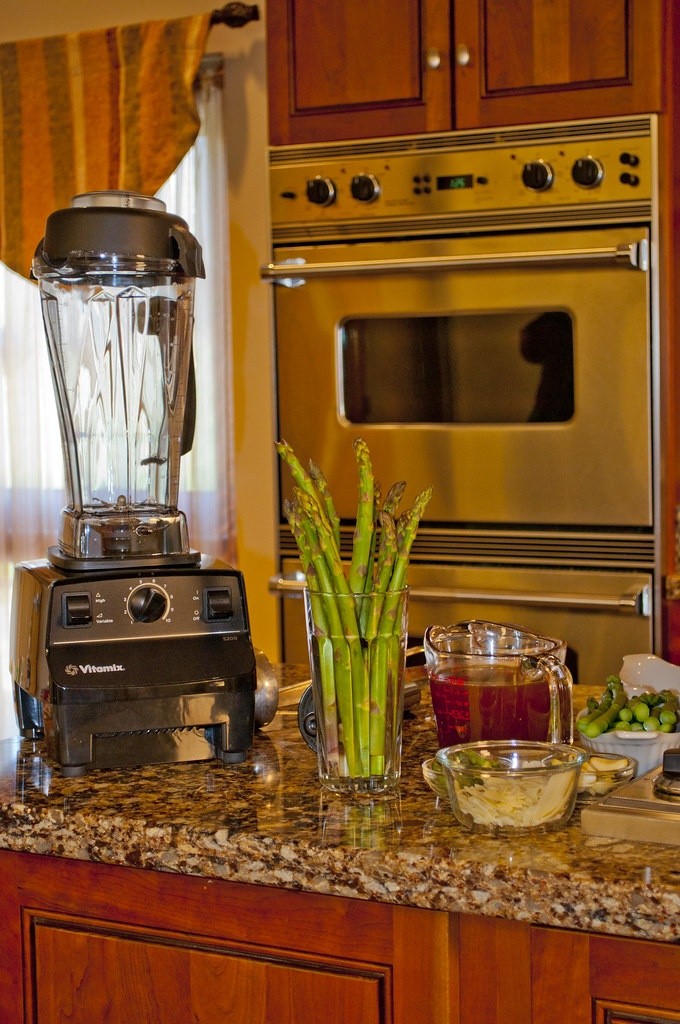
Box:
[619,653,680,706]
[576,750,635,804]
[436,740,588,838]
[576,687,680,777]
[421,758,452,804]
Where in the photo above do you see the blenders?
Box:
[13,188,261,774]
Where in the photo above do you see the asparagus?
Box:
[431,748,498,793]
[577,674,680,739]
[272,438,432,778]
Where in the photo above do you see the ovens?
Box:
[258,115,668,687]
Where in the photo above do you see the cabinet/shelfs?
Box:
[1,853,449,1023]
[453,912,680,1023]
[264,2,679,146]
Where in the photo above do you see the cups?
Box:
[303,585,409,794]
[423,620,575,748]
